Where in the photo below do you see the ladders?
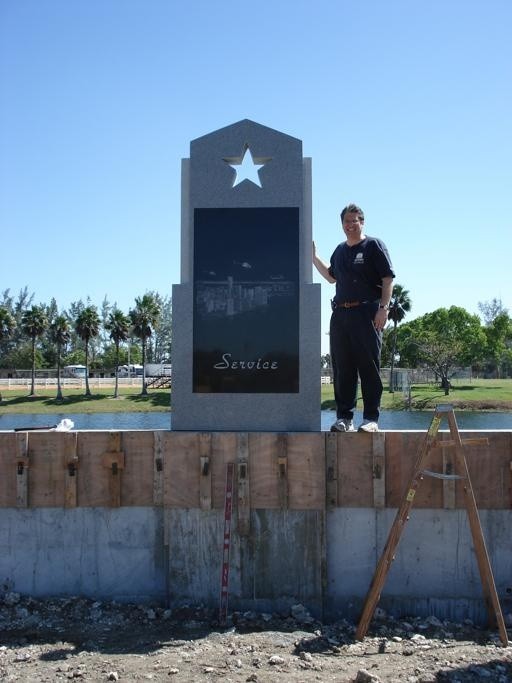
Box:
[353,406,509,648]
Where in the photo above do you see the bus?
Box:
[63,364,89,378]
[118,364,143,378]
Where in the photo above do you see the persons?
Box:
[313,203,395,433]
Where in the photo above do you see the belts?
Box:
[335,302,359,308]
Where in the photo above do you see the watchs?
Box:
[380,306,389,310]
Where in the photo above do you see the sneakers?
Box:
[330,418,378,432]
